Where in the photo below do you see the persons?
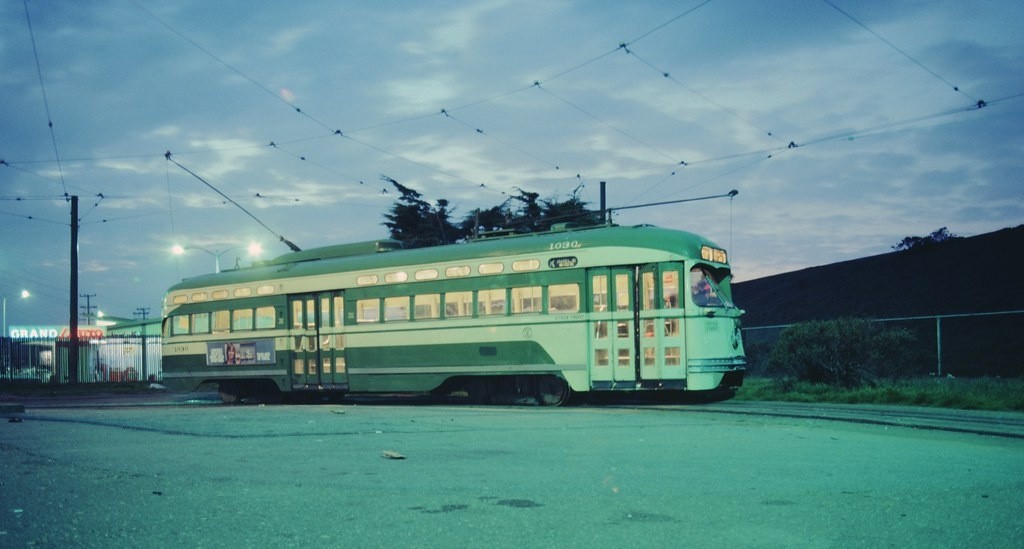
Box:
[222,343,240,364]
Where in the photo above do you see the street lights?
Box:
[2,289,31,368]
[171,239,263,273]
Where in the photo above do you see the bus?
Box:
[161,153,749,407]
